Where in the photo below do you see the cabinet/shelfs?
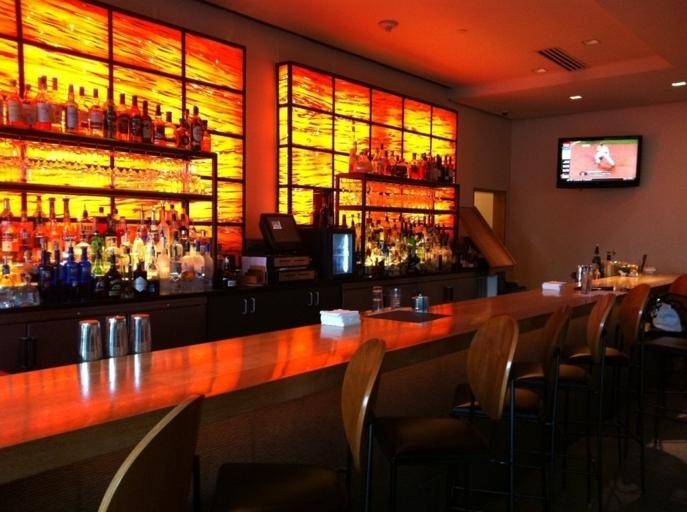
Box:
[206,293,281,342]
[151,305,207,350]
[0,315,129,373]
[344,283,417,308]
[417,277,479,305]
[0,125,218,314]
[281,285,342,329]
[334,172,460,281]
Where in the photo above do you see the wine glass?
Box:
[341,180,444,211]
[115,151,214,196]
[0,138,112,196]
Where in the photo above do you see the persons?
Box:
[594,142,615,166]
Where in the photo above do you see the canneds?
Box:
[106,316,128,357]
[577,264,588,288]
[129,313,151,354]
[77,319,100,360]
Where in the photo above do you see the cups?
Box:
[105,315,129,357]
[130,313,152,353]
[412,295,428,313]
[77,319,103,361]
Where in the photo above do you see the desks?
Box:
[0,276,675,486]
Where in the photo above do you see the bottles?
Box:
[409,151,455,182]
[0,192,134,306]
[390,288,401,309]
[592,245,601,278]
[134,204,239,296]
[611,249,619,276]
[575,263,592,295]
[452,236,478,270]
[372,286,383,313]
[600,261,607,277]
[604,248,612,277]
[349,141,407,176]
[341,212,453,270]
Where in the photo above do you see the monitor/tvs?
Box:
[258,215,303,247]
[557,135,642,189]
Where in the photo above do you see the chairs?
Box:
[636,273,687,426]
[571,283,653,493]
[213,338,386,511]
[512,293,617,511]
[375,314,520,512]
[97,394,203,512]
[450,304,574,511]
